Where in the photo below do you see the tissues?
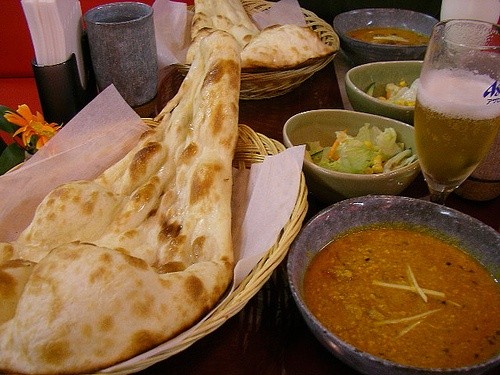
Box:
[20,0,98,127]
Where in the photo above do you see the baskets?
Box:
[175,0,339,100]
[0,118,309,375]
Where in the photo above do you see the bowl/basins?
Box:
[288,194,500,375]
[344,61,424,126]
[282,109,422,204]
[333,7,439,65]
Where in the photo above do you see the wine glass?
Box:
[413,18,500,206]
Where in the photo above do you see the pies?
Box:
[0,28,238,375]
[185,0,336,68]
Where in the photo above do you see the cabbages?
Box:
[305,123,416,174]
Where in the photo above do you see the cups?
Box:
[84,1,157,107]
[440,0,500,182]
